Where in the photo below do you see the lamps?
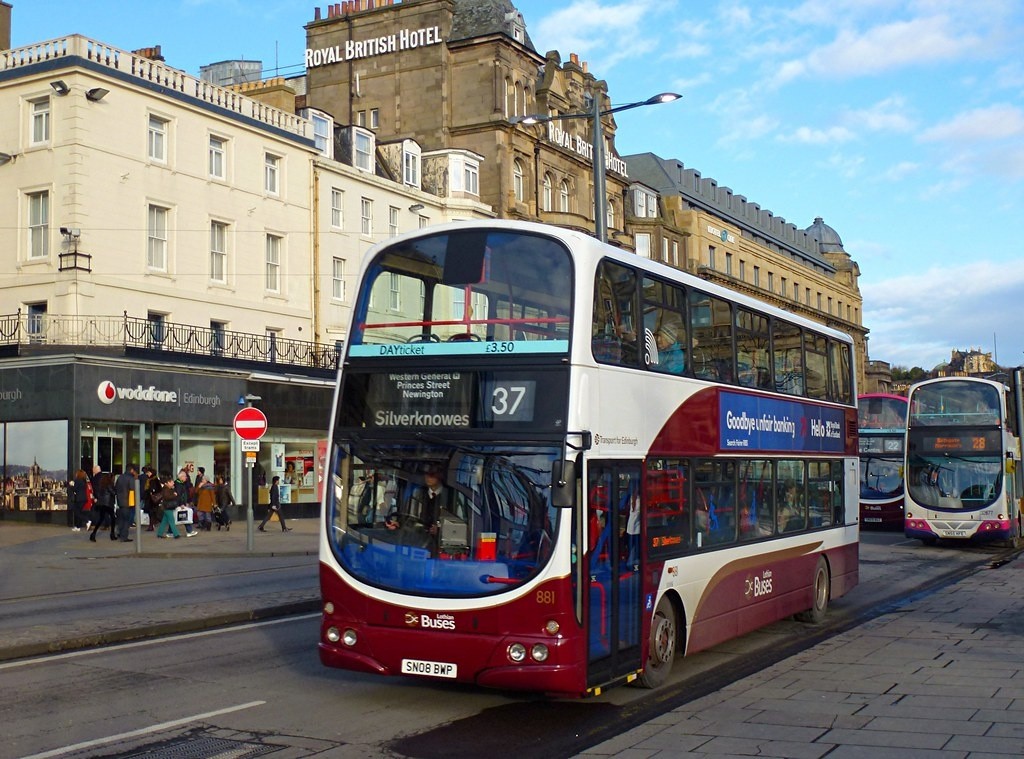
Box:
[50,80,71,95]
[0,153,12,161]
[86,88,110,100]
[409,204,424,211]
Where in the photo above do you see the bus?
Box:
[857,394,909,526]
[318,219,861,697]
[903,376,1024,550]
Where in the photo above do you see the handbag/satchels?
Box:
[173,505,194,525]
[151,492,163,504]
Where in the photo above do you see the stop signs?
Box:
[231,404,268,445]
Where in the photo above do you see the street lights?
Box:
[518,84,681,249]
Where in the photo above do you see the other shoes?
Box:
[72,526,81,531]
[111,536,118,540]
[187,531,198,537]
[282,527,293,532]
[158,535,166,538]
[86,520,92,531]
[145,528,155,531]
[90,536,96,541]
[122,538,133,542]
[175,534,181,539]
[166,533,174,538]
[258,525,267,532]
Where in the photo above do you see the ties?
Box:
[431,492,436,498]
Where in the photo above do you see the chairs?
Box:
[592,334,804,395]
[408,334,483,343]
[592,468,836,564]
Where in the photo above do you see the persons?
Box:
[386,460,453,546]
[286,462,296,477]
[858,418,870,429]
[69,466,235,542]
[258,476,293,532]
[759,479,832,539]
[618,322,684,373]
[355,476,394,524]
[627,483,641,567]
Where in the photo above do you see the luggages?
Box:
[212,506,232,531]
